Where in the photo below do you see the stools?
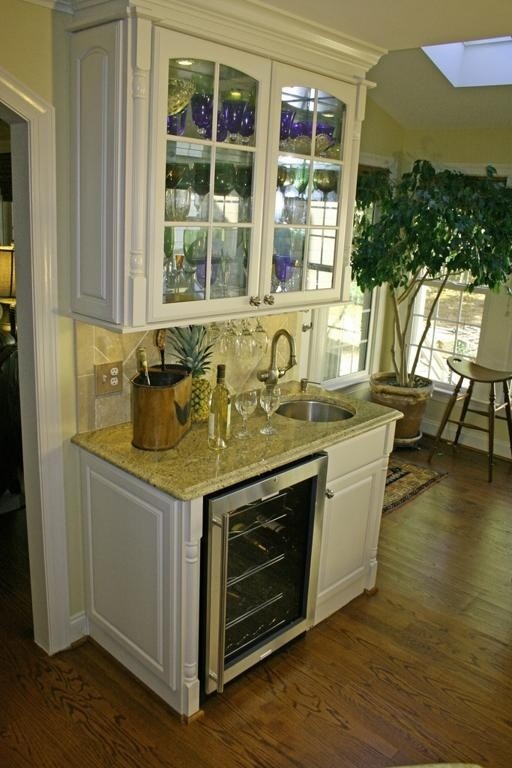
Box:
[425,354,512,483]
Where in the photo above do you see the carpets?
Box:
[381,451,449,517]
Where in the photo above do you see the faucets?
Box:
[256,330,297,393]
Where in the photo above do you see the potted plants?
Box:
[340,155,512,451]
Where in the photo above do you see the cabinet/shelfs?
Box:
[67,424,393,724]
[51,1,389,339]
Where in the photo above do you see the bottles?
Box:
[135,347,152,387]
[207,364,231,451]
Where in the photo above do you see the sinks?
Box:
[275,395,355,422]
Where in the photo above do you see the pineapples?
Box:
[164,324,213,424]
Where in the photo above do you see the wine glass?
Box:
[165,71,337,305]
[235,386,281,440]
[213,318,271,361]
